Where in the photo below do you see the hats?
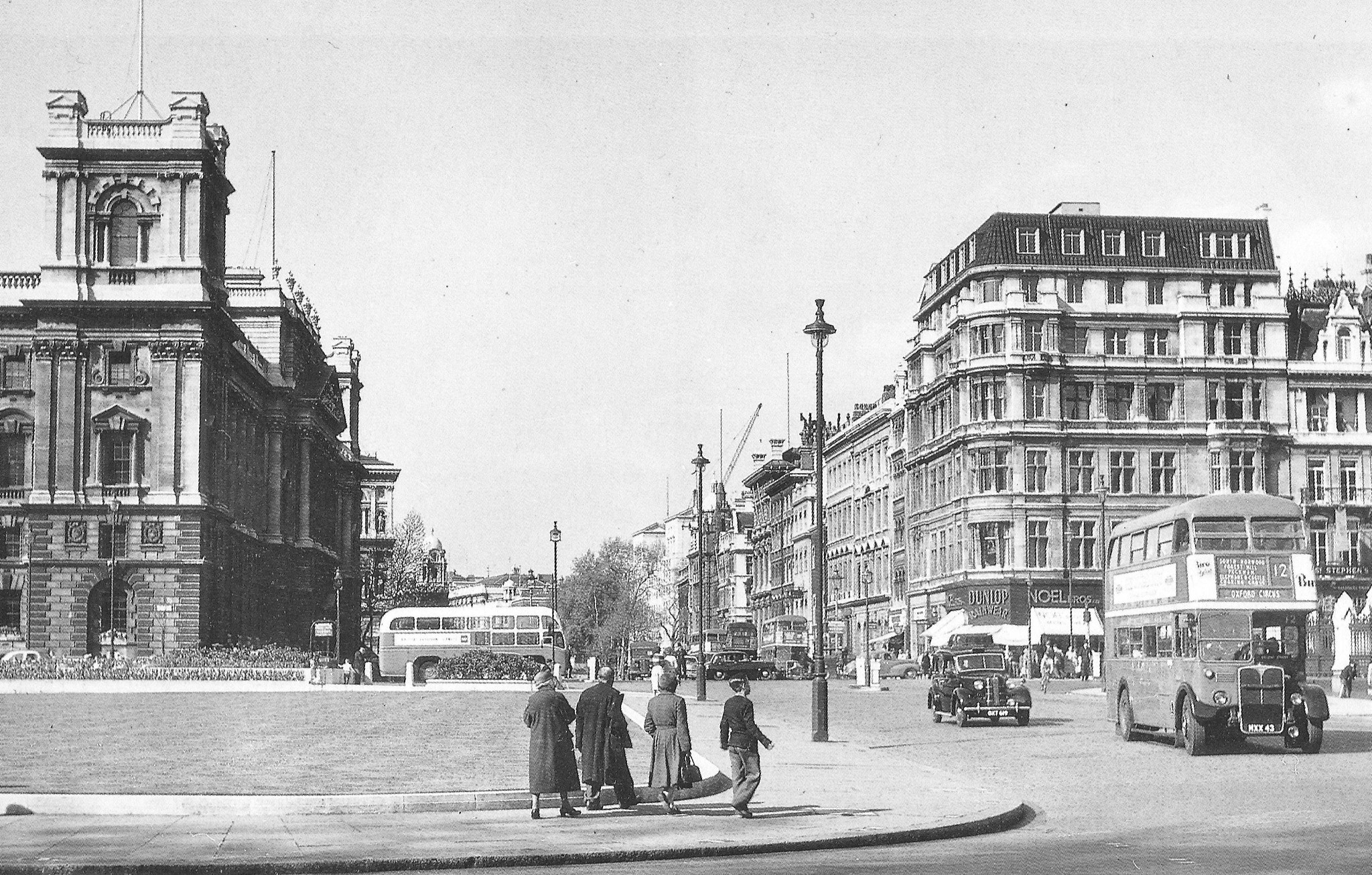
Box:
[534,671,553,684]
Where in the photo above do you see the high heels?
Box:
[560,806,581,817]
[532,809,539,819]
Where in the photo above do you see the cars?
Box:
[705,650,778,680]
[926,651,1033,727]
[1,651,50,669]
[844,650,919,679]
[680,655,698,681]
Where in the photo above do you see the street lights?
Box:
[856,565,875,687]
[803,298,838,742]
[333,567,344,665]
[23,528,36,649]
[108,492,123,662]
[689,444,709,702]
[830,569,843,654]
[548,521,562,672]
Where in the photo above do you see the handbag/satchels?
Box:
[681,755,701,784]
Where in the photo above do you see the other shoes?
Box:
[732,803,753,819]
[666,808,682,815]
[621,795,641,808]
[658,793,671,812]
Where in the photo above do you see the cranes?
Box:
[720,403,766,494]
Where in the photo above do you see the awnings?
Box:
[921,606,1104,645]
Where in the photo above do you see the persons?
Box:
[523,671,581,818]
[1341,661,1357,698]
[338,658,356,684]
[644,673,691,816]
[576,667,637,809]
[353,647,366,685]
[651,644,687,694]
[720,672,774,818]
[1003,643,1092,690]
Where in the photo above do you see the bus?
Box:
[378,606,567,682]
[627,640,659,679]
[1104,491,1330,755]
[759,616,810,678]
[687,623,756,657]
